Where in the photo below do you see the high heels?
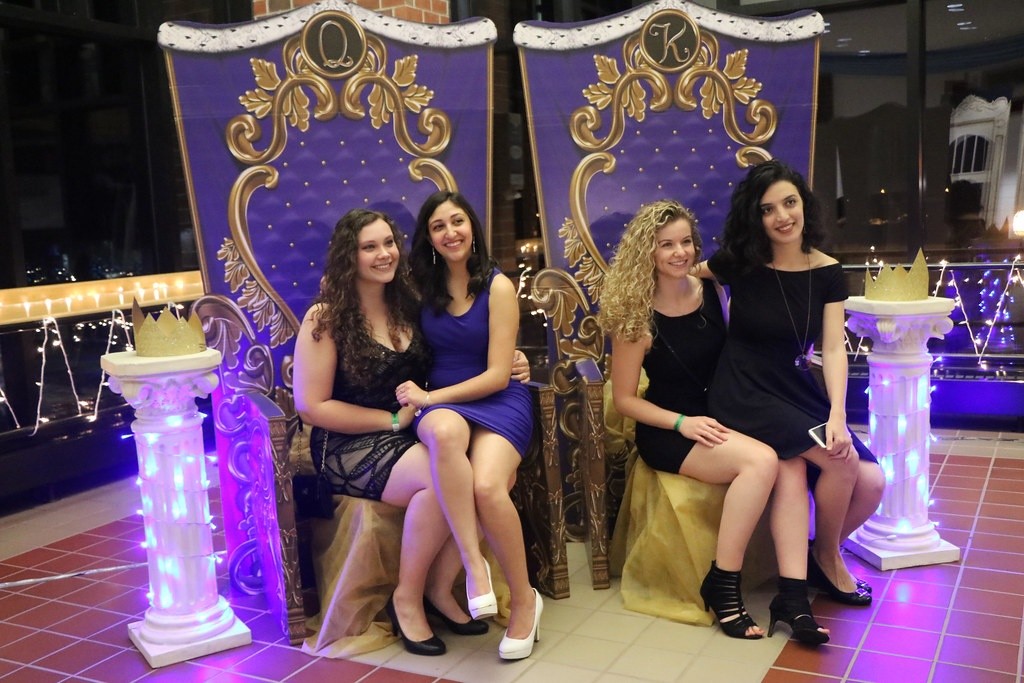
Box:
[423,596,489,635]
[498,588,544,660]
[699,560,762,640]
[466,558,498,620]
[766,576,830,644]
[807,541,873,604]
[386,590,446,656]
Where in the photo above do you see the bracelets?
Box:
[674,414,687,433]
[391,414,400,432]
[415,392,431,416]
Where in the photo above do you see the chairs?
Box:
[156,0,827,648]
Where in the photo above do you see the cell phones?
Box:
[809,422,829,448]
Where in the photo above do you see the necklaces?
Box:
[772,253,812,371]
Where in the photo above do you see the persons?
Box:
[689,159,886,606]
[596,198,830,644]
[319,191,543,659]
[292,207,530,657]
